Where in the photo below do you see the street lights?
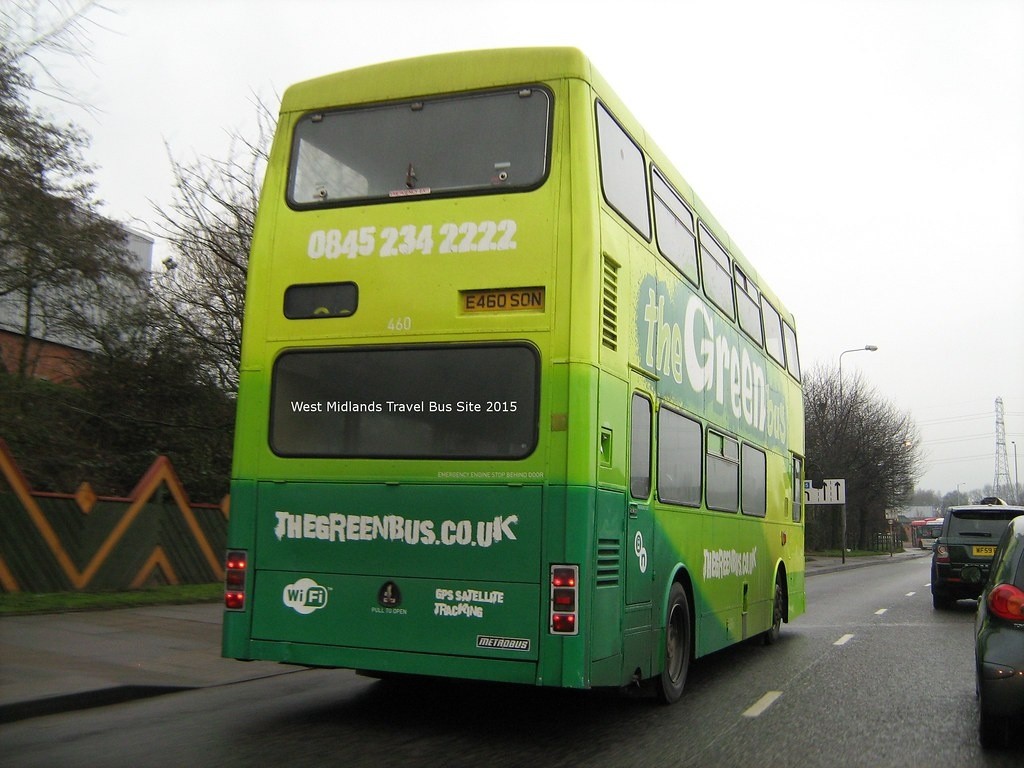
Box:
[892,441,912,518]
[839,344,878,428]
[957,482,966,506]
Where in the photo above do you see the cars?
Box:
[975,513,1024,751]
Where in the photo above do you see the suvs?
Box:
[930,495,1024,609]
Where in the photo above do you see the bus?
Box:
[911,518,935,548]
[920,517,944,553]
[223,49,807,693]
